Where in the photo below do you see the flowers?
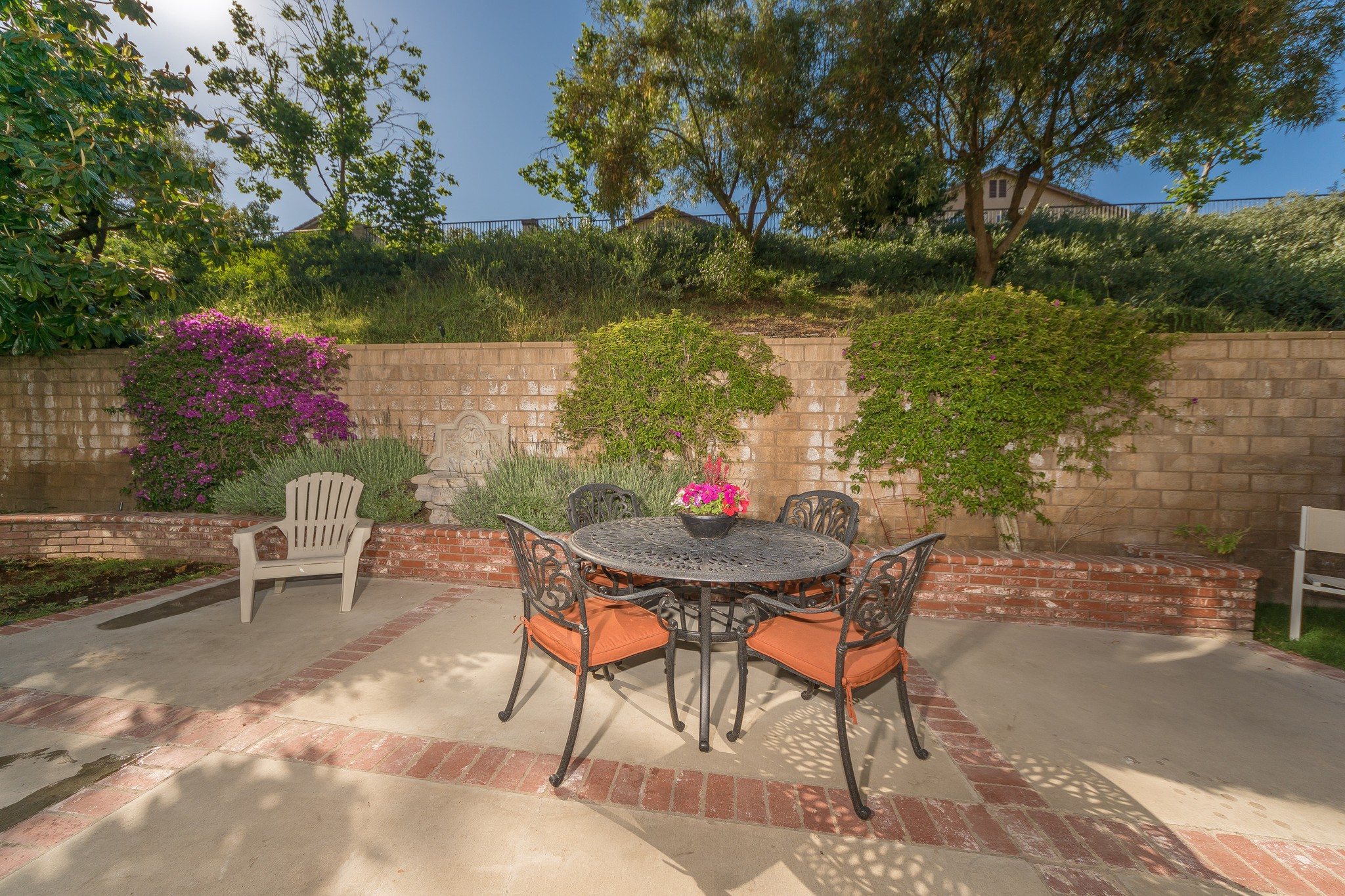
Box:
[671,481,750,517]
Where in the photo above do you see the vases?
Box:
[677,511,738,540]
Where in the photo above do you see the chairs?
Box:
[1289,506,1345,641]
[742,490,859,617]
[727,533,947,821]
[567,483,677,611]
[232,472,374,625]
[496,513,685,787]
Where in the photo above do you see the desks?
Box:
[567,518,854,751]
